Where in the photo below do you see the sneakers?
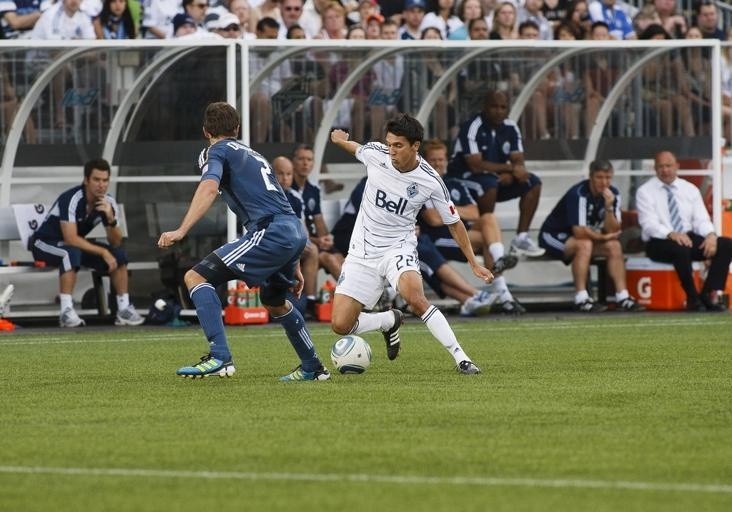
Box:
[59,306,86,328]
[460,290,526,317]
[510,235,546,257]
[457,359,480,374]
[279,364,330,381]
[114,303,145,326]
[382,308,404,360]
[574,296,608,312]
[493,254,518,273]
[176,355,235,379]
[616,295,646,311]
[688,287,729,313]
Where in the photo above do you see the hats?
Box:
[219,14,240,30]
[366,13,384,23]
[174,13,196,33]
[404,0,425,10]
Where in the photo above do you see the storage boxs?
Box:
[625,258,706,311]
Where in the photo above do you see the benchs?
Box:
[515,253,607,311]
[1,262,225,318]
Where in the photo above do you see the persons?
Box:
[636,150,732,311]
[292,142,345,279]
[157,101,331,382]
[539,159,648,314]
[0,0,732,149]
[31,157,147,329]
[331,175,499,317]
[423,139,528,315]
[446,90,546,259]
[331,115,495,375]
[272,156,319,305]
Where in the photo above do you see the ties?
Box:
[665,185,684,233]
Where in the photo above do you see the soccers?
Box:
[330,335,371,374]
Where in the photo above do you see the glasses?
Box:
[221,26,237,32]
[199,4,209,8]
[287,6,299,11]
